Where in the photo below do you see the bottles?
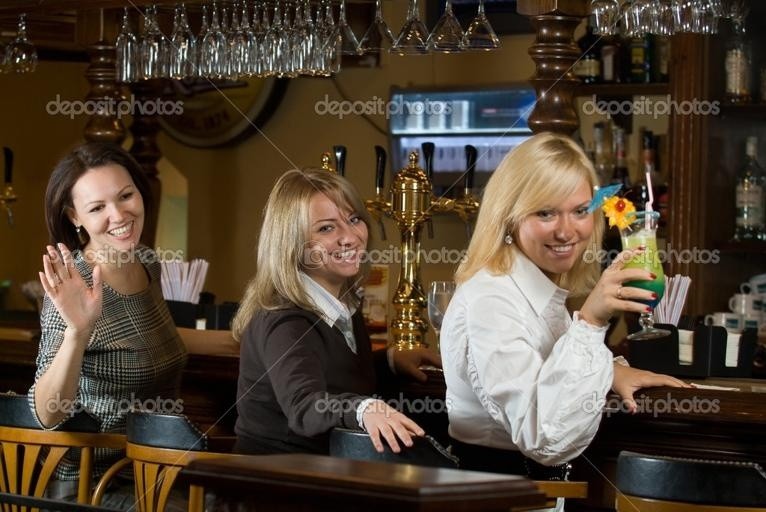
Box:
[573,27,669,84]
[734,136,766,242]
[723,0,756,105]
[591,120,667,233]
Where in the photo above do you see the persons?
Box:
[445,130,692,480]
[231,167,445,457]
[27,139,190,512]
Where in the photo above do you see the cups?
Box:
[704,277,766,332]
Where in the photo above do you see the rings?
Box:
[617,285,622,300]
[49,273,61,289]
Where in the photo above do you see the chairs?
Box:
[126,412,259,512]
[330,426,588,512]
[613,451,766,511]
[0,393,131,512]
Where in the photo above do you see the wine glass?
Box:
[617,211,673,341]
[5,9,39,73]
[116,0,500,83]
[428,280,456,371]
[588,0,719,37]
